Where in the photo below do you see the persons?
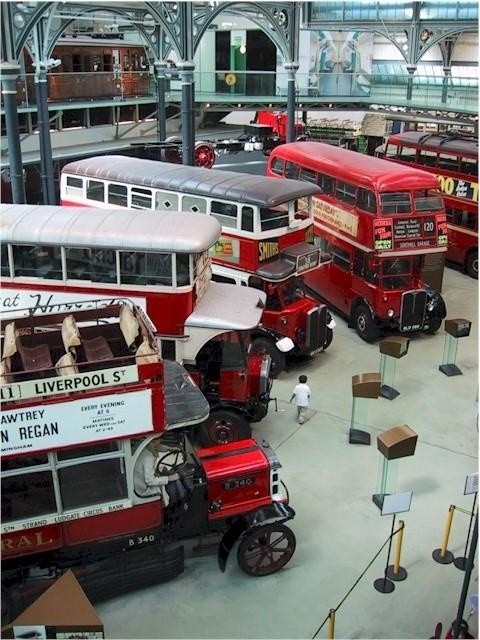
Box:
[289,375,311,424]
[134,437,187,507]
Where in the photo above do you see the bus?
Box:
[0,294,301,600]
[0,202,278,448]
[376,130,479,280]
[265,142,450,343]
[59,151,331,380]
